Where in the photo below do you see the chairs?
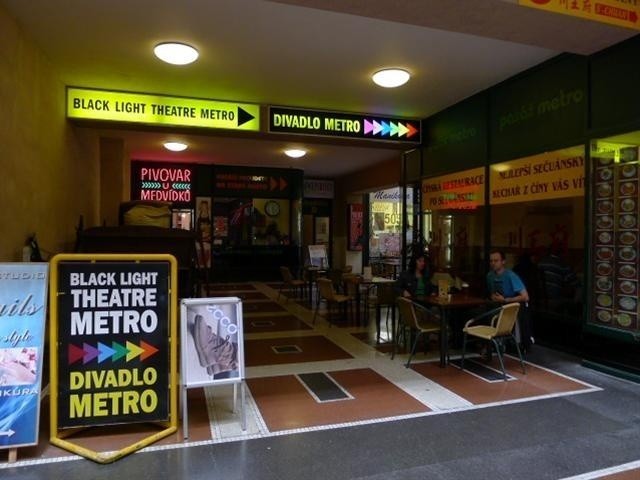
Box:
[280,267,526,381]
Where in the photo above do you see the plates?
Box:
[596,165,637,327]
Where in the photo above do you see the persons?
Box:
[395,249,442,321]
[481,250,530,357]
[192,314,240,376]
[2,361,38,385]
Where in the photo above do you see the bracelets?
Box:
[503,296,508,304]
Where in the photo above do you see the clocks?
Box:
[264,201,281,216]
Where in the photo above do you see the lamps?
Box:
[370,67,410,90]
[153,43,198,66]
